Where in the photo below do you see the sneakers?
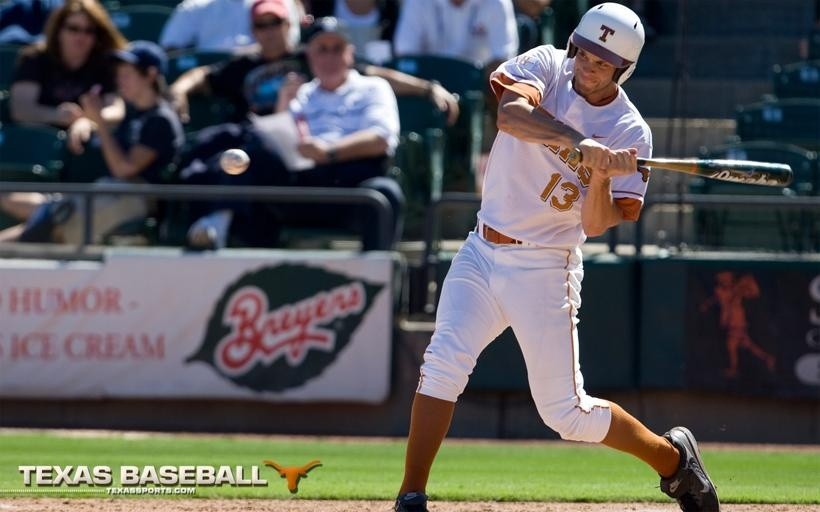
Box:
[659,426,722,512]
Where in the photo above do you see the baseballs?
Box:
[220,150,251,175]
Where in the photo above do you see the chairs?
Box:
[689,28,820,252]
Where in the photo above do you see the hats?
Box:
[248,0,292,23]
[567,3,648,86]
[109,42,171,74]
[305,14,355,42]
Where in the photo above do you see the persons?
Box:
[0,1,552,250]
[393,1,718,512]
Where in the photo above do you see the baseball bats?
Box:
[568,146,794,187]
